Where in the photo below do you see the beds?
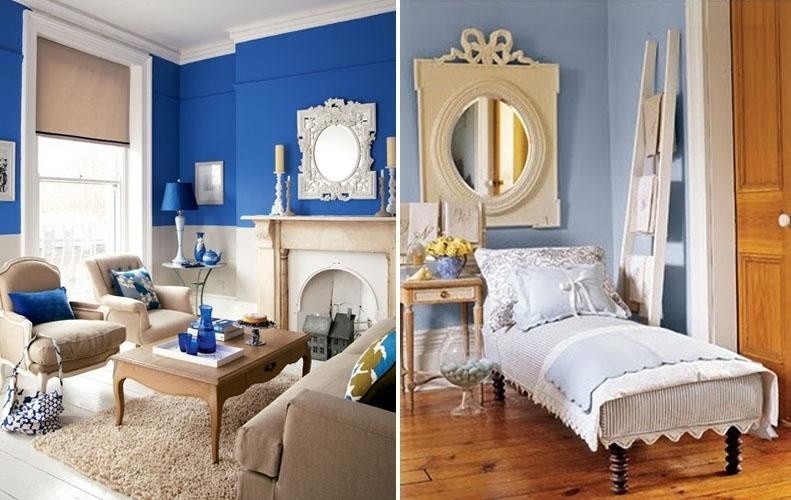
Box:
[474,245,780,495]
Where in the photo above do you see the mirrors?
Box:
[296,97,378,201]
[413,29,561,229]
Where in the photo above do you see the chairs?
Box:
[0,257,126,397]
[84,255,199,347]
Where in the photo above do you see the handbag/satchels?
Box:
[0,332,64,436]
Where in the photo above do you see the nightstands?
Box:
[401,277,483,412]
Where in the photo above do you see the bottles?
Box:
[194,232,206,262]
[196,304,216,354]
[405,231,426,280]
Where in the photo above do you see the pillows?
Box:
[109,266,163,310]
[343,326,395,412]
[8,287,76,326]
[474,245,633,332]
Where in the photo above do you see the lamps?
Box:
[160,179,199,265]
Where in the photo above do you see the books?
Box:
[186,316,245,342]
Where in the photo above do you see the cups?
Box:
[184,338,200,356]
[178,332,192,352]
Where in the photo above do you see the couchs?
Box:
[235,318,396,500]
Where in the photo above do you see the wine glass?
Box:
[439,324,494,419]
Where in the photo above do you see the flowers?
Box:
[426,234,475,258]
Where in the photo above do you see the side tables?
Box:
[161,263,226,319]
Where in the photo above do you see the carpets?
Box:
[32,371,301,500]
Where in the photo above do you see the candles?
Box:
[288,175,290,181]
[386,137,396,167]
[381,169,384,177]
[275,144,284,173]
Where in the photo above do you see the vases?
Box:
[424,255,466,279]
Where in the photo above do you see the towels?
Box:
[636,175,655,234]
[625,253,663,320]
[642,91,679,158]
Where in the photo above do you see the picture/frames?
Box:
[194,162,224,205]
[0,139,15,201]
